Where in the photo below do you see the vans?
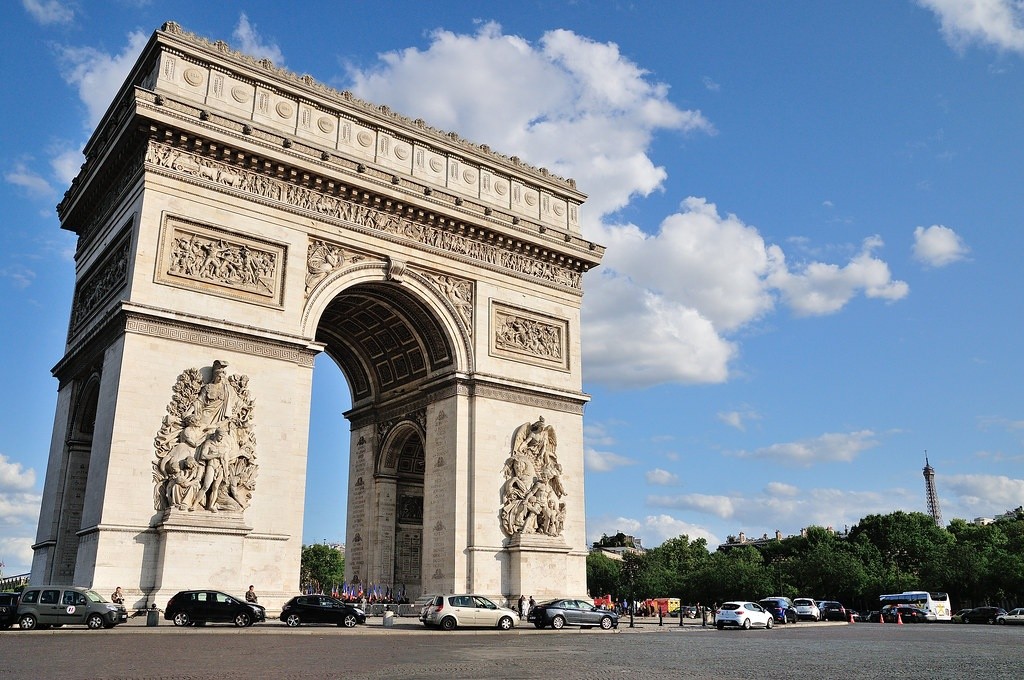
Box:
[0,592,20,630]
[16,585,128,630]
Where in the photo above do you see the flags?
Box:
[307,581,406,603]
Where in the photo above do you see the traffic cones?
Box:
[880,614,885,624]
[850,614,855,624]
[897,614,904,625]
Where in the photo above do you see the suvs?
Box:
[758,599,797,625]
[961,607,1007,624]
[794,598,821,622]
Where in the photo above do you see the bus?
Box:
[646,598,682,618]
[879,591,952,622]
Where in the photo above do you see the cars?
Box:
[163,589,267,628]
[419,598,482,627]
[716,601,774,630]
[670,605,712,618]
[280,594,366,628]
[996,608,1024,624]
[866,607,928,624]
[845,609,879,624]
[766,597,792,604]
[818,602,846,622]
[426,594,521,631]
[951,608,973,624]
[526,599,620,631]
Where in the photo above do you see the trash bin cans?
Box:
[147,611,159,627]
[383,611,393,626]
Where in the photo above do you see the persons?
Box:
[518,595,537,620]
[245,585,258,603]
[112,587,124,604]
[307,247,359,294]
[513,319,560,357]
[168,360,254,513]
[426,276,473,335]
[520,423,568,536]
[601,598,718,618]
[174,235,257,285]
